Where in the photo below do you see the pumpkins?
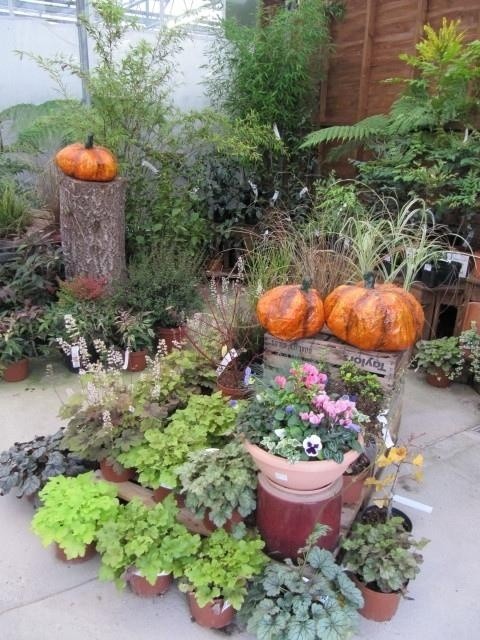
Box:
[325,272,426,354]
[54,131,120,182]
[255,278,325,345]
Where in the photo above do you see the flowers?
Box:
[229,356,428,505]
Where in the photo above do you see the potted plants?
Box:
[0,234,284,632]
[336,515,433,623]
[400,318,479,400]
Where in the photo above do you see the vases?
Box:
[242,468,415,565]
